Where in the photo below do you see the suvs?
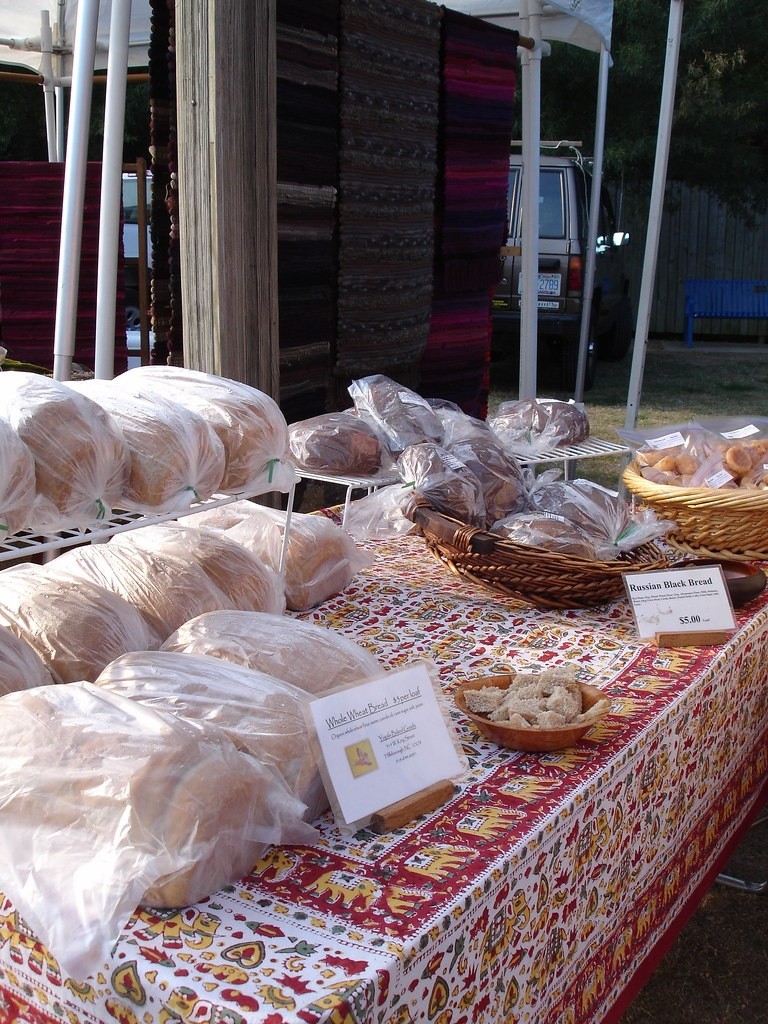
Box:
[489,136,638,395]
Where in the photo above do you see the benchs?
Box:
[682,277,768,347]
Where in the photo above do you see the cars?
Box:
[118,170,156,368]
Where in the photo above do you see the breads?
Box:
[0,362,768,912]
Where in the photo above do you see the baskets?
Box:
[400,486,670,610]
[621,450,768,561]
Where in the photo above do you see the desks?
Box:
[0,504,768,1024]
